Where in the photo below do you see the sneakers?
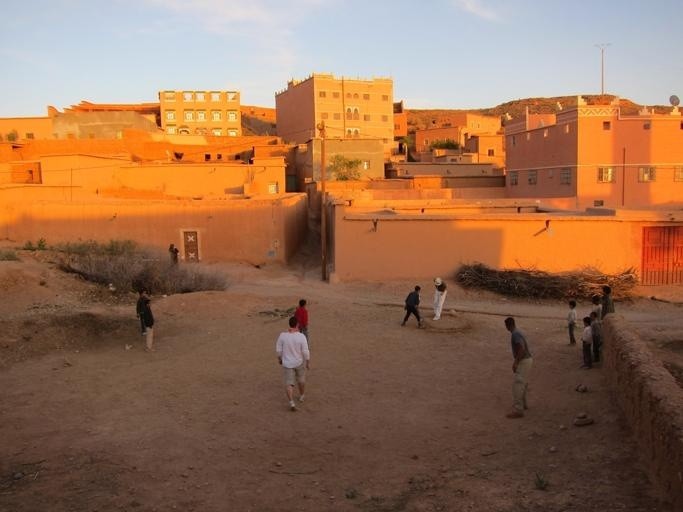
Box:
[507,412,523,417]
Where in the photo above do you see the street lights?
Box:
[315,118,328,281]
[593,40,612,103]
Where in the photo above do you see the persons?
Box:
[135,290,150,335]
[431,278,447,320]
[502,315,532,418]
[167,243,180,271]
[274,317,311,407]
[563,281,616,369]
[142,300,157,350]
[293,300,309,343]
[400,285,422,328]
